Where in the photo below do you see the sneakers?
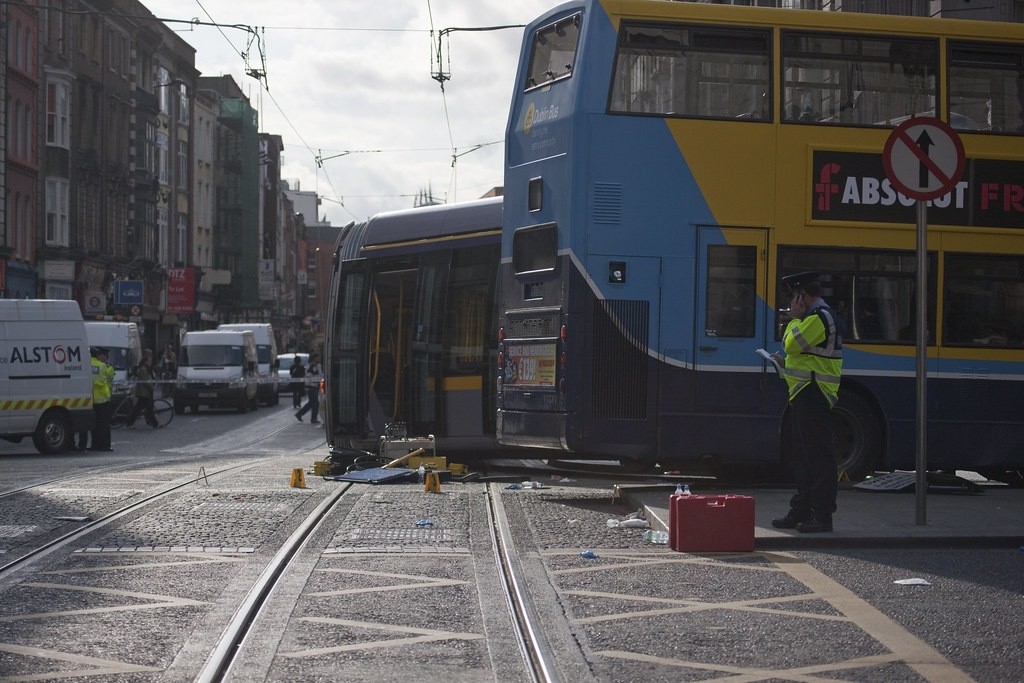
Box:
[771,509,807,527]
[795,517,832,533]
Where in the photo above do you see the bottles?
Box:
[418,463,425,484]
[683,485,691,494]
[522,481,544,489]
[675,483,683,494]
[642,530,668,543]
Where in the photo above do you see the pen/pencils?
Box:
[771,351,779,357]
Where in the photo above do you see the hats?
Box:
[89,345,109,355]
[782,271,820,296]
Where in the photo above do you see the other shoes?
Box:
[311,420,321,423]
[295,414,303,422]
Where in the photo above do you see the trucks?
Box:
[0,298,95,453]
[172,329,260,414]
[217,322,282,407]
[83,321,143,420]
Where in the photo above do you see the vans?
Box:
[276,353,321,392]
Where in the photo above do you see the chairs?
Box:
[859,297,897,341]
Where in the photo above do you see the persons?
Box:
[771,270,842,533]
[78,345,115,451]
[163,345,176,373]
[289,355,305,407]
[127,348,164,428]
[295,352,322,423]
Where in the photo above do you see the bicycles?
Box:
[108,386,174,430]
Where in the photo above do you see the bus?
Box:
[313,192,619,461]
[496,0,1023,491]
